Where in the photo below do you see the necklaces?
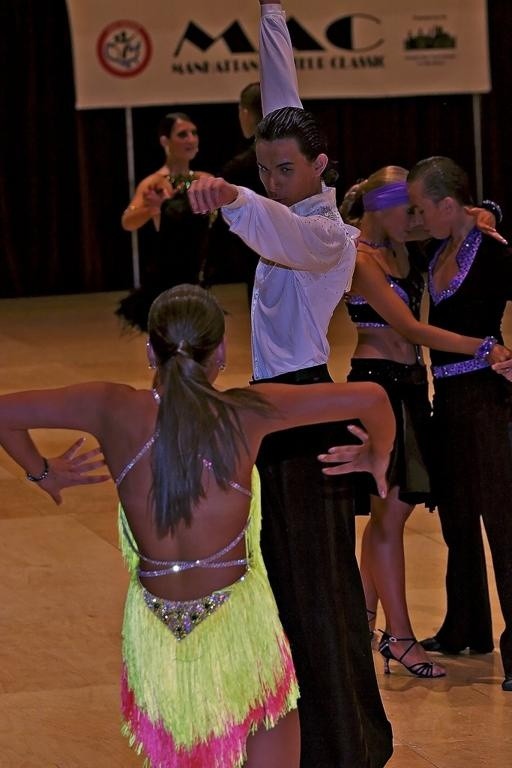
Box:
[357,232,398,264]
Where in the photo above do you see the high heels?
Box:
[377,628,447,679]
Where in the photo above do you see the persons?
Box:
[216,80,269,322]
[184,1,396,768]
[111,110,217,333]
[1,285,409,768]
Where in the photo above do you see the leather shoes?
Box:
[502,677,512,691]
[420,637,488,656]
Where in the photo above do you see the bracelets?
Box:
[475,334,496,366]
[24,456,49,483]
